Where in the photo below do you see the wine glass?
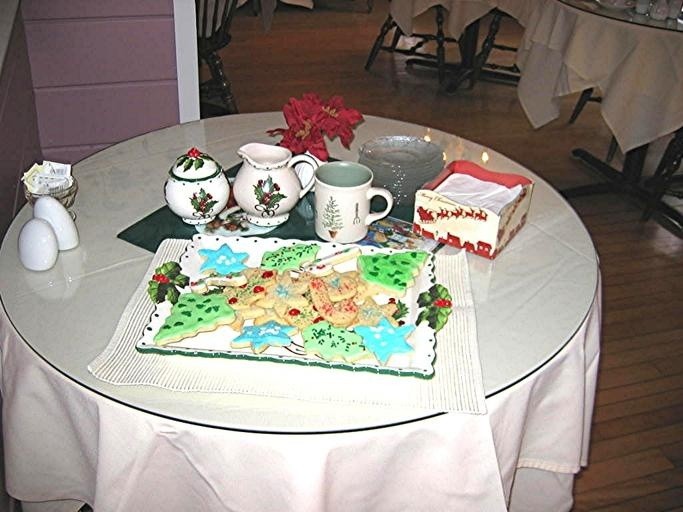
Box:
[24,174,79,223]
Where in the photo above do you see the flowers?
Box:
[265,92,364,161]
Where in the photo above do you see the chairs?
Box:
[195,1,241,113]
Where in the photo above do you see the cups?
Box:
[313,161,395,245]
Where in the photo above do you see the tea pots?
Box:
[230,139,319,227]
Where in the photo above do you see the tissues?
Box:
[413,158,535,261]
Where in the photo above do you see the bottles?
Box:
[595,0,683,22]
[164,146,230,227]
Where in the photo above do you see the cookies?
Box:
[152,243,429,367]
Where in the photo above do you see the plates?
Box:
[357,133,448,212]
[134,229,453,381]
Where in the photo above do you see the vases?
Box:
[295,152,329,194]
